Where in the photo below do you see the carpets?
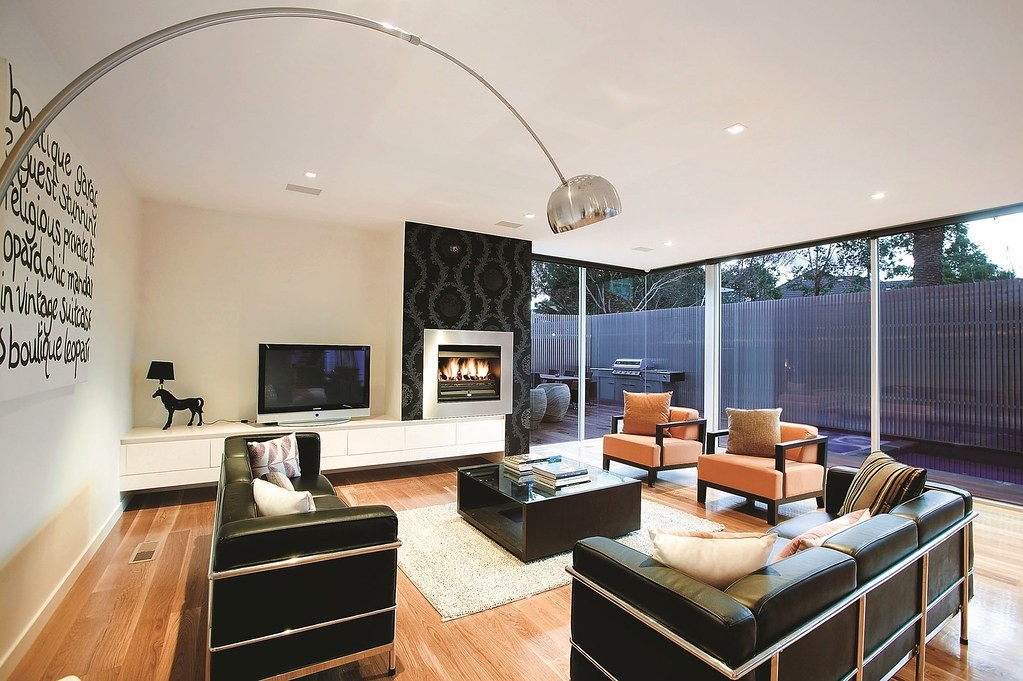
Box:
[395,499,726,622]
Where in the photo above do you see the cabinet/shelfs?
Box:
[597,370,686,406]
[120,412,506,492]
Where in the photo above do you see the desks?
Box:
[540,374,591,380]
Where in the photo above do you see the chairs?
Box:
[602,408,707,488]
[697,422,828,525]
[775,391,842,426]
[529,369,593,431]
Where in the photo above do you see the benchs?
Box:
[565,465,978,681]
[206,433,403,681]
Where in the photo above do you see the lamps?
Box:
[0,7,624,234]
[146,361,204,430]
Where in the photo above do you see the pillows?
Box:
[261,473,295,491]
[648,528,777,592]
[787,382,815,395]
[251,477,316,518]
[247,432,302,479]
[837,451,927,518]
[725,408,782,458]
[621,390,673,439]
[771,508,870,565]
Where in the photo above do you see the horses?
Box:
[152,389,205,430]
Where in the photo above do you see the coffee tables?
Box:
[457,457,642,562]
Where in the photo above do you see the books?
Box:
[532,482,591,498]
[504,453,591,489]
[503,468,534,486]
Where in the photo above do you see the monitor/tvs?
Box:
[257,343,370,427]
